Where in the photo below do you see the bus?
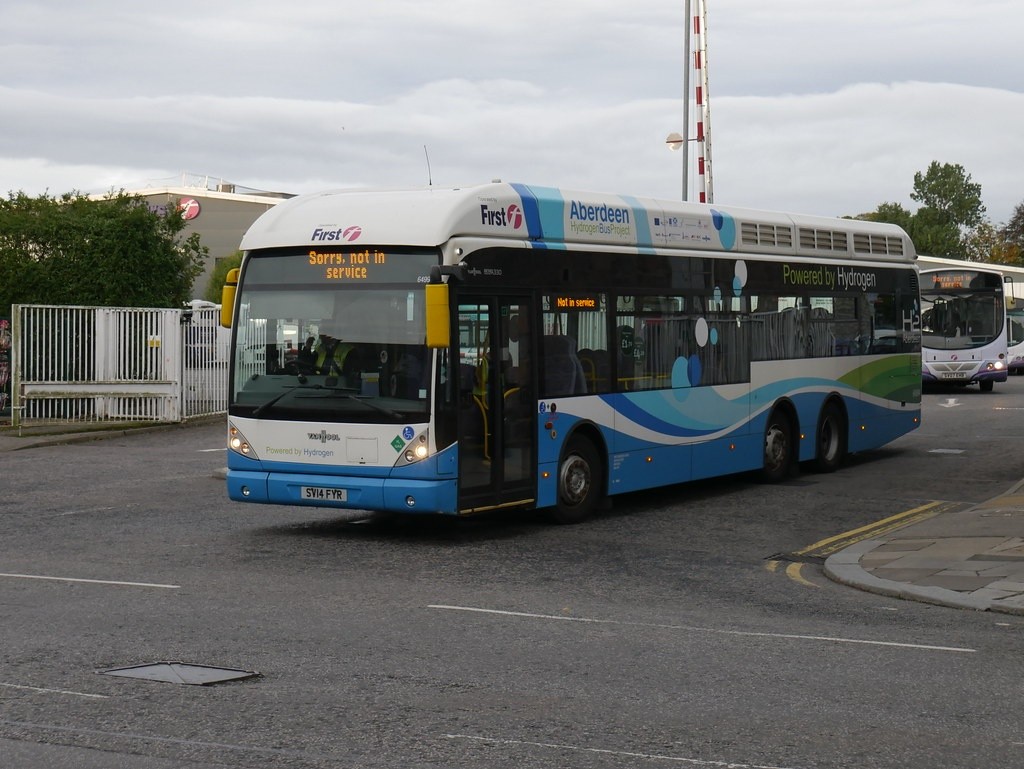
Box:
[220,181,923,523]
[835,266,1024,393]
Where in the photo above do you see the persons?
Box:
[300,318,355,377]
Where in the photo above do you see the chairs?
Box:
[460,335,608,395]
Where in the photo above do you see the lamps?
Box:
[180,306,193,325]
